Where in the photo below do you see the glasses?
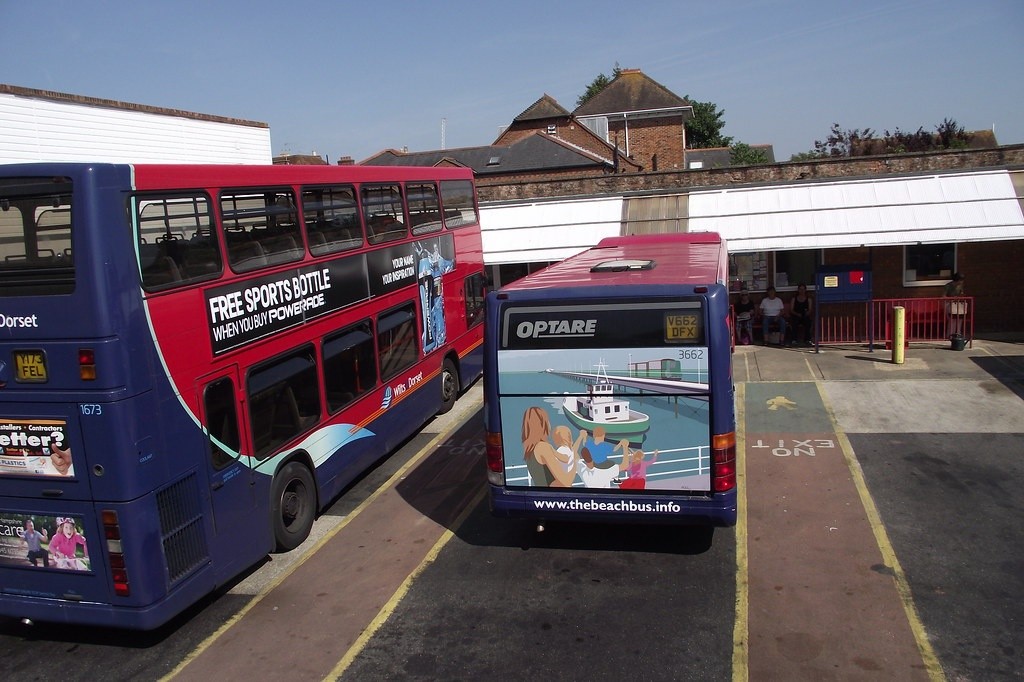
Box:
[741,294,748,296]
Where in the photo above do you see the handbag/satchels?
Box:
[736,312,751,321]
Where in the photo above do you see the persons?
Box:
[944,272,967,339]
[734,282,813,348]
[21,520,48,566]
[49,516,88,572]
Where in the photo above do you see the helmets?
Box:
[57,517,76,527]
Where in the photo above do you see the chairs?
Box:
[270,379,318,439]
[306,374,354,414]
[0,210,463,298]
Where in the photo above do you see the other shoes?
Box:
[786,341,793,347]
[749,338,754,344]
[806,340,815,346]
[736,338,742,344]
[763,340,769,346]
[779,341,785,346]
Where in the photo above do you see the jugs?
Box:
[949,334,973,350]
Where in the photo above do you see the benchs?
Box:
[1,544,41,566]
[751,303,796,328]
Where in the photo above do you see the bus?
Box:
[0,162,490,635]
[482,231,739,552]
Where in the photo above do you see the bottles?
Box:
[431,263,446,344]
[418,250,436,351]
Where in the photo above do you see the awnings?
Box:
[397,170,1024,267]
